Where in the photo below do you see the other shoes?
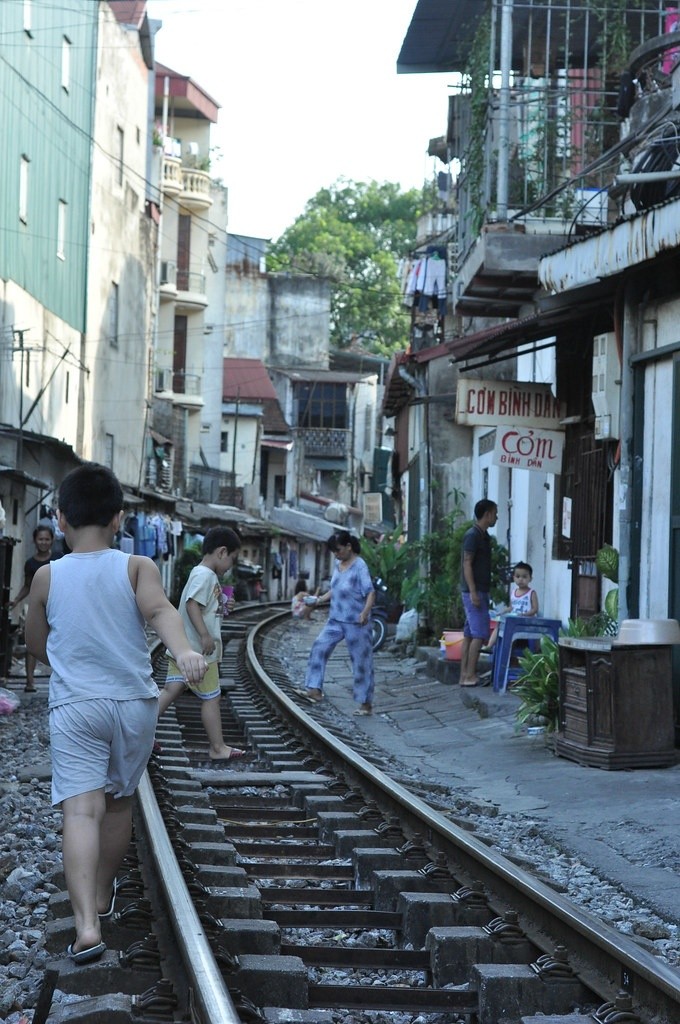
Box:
[24,685,38,691]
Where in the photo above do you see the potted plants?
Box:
[354,521,619,740]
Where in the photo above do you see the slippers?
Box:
[68,937,106,961]
[96,876,118,917]
[292,688,317,703]
[212,747,246,763]
[461,678,490,688]
[353,708,370,715]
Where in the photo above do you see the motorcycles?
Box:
[370,573,396,651]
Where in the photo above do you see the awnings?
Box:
[405,292,623,371]
[397,0,492,74]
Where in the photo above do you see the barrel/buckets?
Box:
[441,632,466,660]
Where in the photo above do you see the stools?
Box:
[490,615,561,693]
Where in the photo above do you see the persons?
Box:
[25,462,209,963]
[482,563,539,651]
[9,525,54,692]
[292,530,376,716]
[255,581,267,601]
[458,499,499,687]
[158,527,250,762]
[292,575,332,623]
